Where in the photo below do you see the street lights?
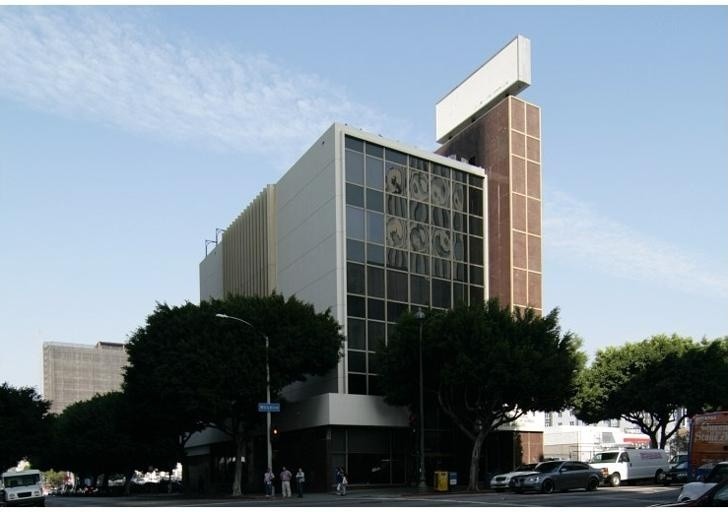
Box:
[215,312,276,498]
[414,308,426,489]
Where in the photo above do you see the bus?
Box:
[687,410,728,484]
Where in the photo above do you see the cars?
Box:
[659,454,728,506]
[489,459,604,495]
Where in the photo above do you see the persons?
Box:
[264,467,276,499]
[279,465,292,499]
[338,464,344,476]
[340,473,348,497]
[296,467,305,498]
[334,467,342,496]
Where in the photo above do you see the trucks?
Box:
[587,448,672,487]
[0,469,45,507]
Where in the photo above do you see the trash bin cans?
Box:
[433,469,449,492]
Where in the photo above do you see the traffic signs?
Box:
[258,402,281,413]
